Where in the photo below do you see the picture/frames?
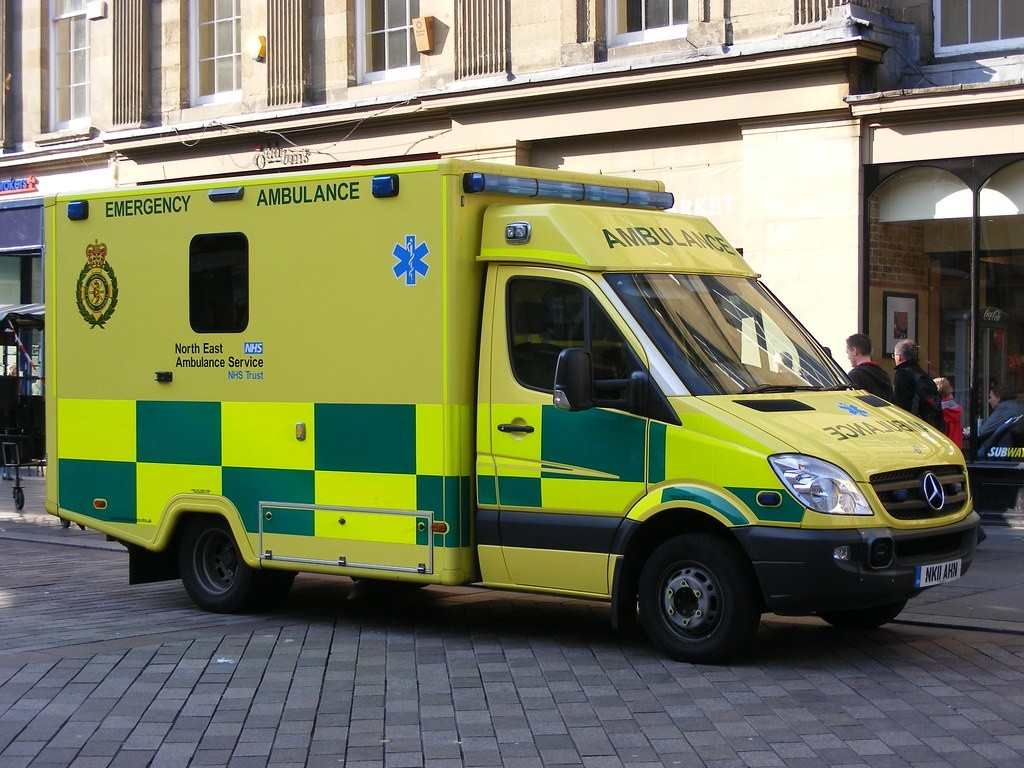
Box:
[882,291,919,359]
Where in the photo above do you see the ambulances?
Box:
[44,158,987,662]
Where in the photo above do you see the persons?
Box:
[892,339,945,432]
[846,333,893,401]
[978,381,1024,441]
[933,377,988,546]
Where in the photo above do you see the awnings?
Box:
[0,303,46,322]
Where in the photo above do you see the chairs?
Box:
[514,339,563,394]
[602,346,636,399]
